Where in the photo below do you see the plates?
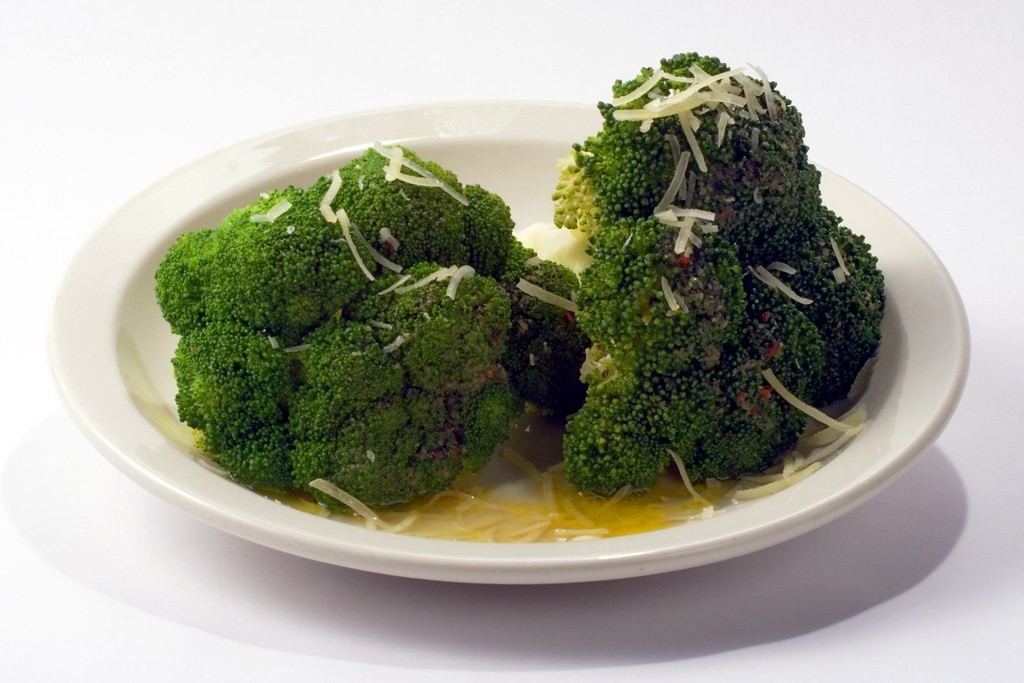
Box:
[45,98,971,586]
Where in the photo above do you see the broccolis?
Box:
[551,54,888,504]
[156,147,582,512]
